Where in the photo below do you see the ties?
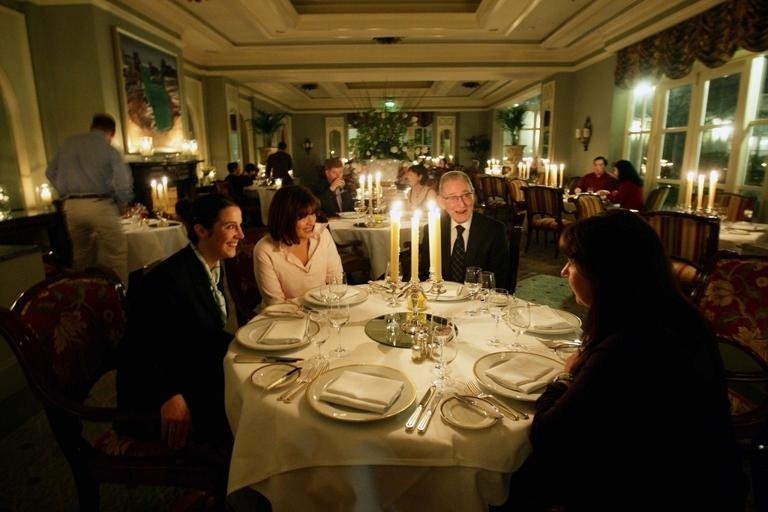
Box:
[449,225,466,284]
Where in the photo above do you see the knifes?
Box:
[406,383,436,434]
[234,353,305,365]
[418,385,444,434]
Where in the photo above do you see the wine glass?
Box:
[428,309,458,386]
[463,268,531,347]
[675,203,728,231]
[384,260,403,300]
[317,268,351,358]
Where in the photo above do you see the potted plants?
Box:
[497,102,529,163]
[250,107,289,164]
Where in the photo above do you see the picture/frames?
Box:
[113,25,190,157]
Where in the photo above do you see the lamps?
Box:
[575,116,592,151]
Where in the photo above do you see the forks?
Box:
[284,358,333,403]
[462,380,519,421]
[467,381,528,421]
[275,358,317,401]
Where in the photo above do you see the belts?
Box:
[68,194,112,199]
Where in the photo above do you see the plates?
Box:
[250,363,300,391]
[437,395,503,432]
[419,280,467,301]
[332,178,401,226]
[303,284,370,305]
[505,306,583,335]
[471,349,575,404]
[235,315,320,352]
[307,363,417,423]
[262,303,299,316]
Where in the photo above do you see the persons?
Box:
[45,114,134,294]
[226,162,261,224]
[574,157,643,210]
[403,171,509,294]
[253,185,344,311]
[402,166,437,210]
[116,194,271,512]
[511,209,744,512]
[266,142,294,185]
[319,159,357,216]
[441,159,448,169]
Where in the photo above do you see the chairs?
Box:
[690,253,767,432]
[703,330,766,510]
[28,160,768,305]
[1,266,227,508]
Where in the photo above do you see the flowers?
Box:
[343,93,431,159]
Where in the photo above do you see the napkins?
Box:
[320,369,404,416]
[484,353,560,395]
[257,318,310,346]
[517,305,573,330]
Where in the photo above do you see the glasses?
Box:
[440,192,473,203]
